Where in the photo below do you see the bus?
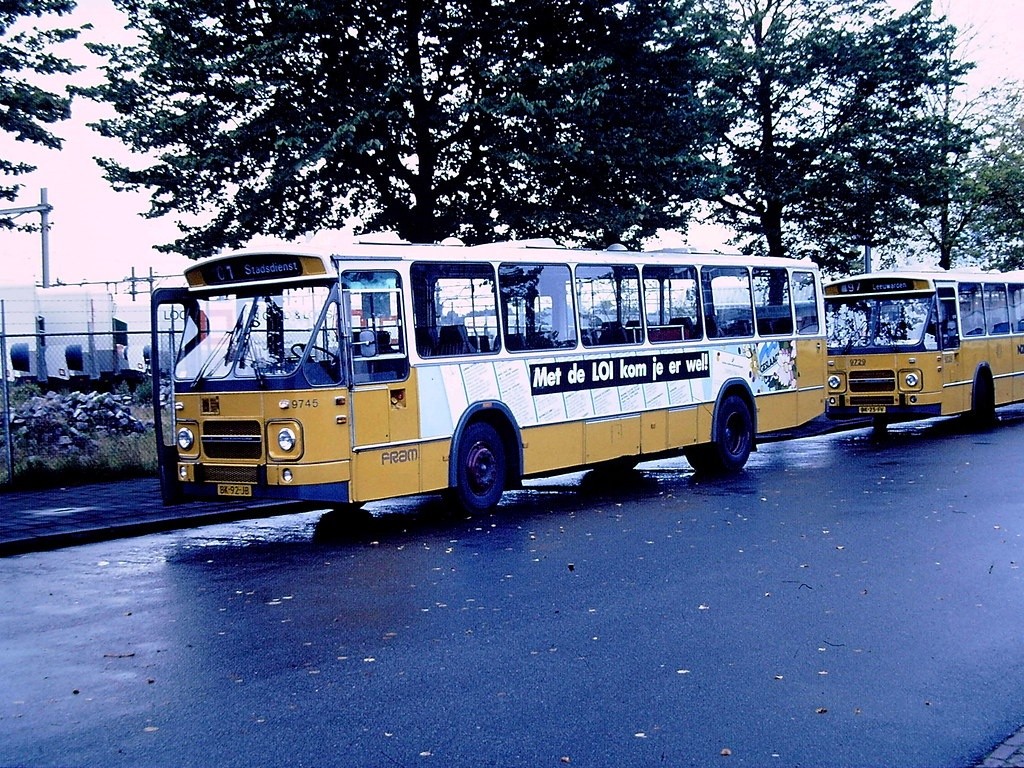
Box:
[824,267,1024,428]
[152,231,828,515]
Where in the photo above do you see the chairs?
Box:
[352,315,791,370]
